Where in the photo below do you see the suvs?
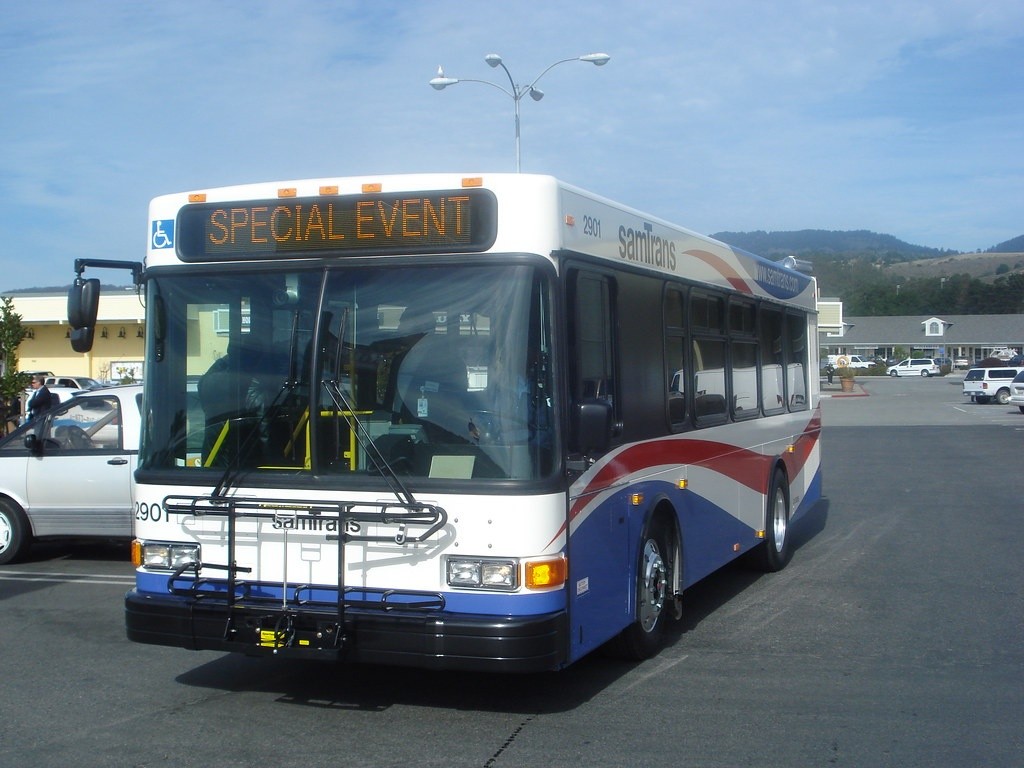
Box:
[1006,369,1024,414]
[961,366,1024,404]
[886,358,941,378]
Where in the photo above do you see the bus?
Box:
[63,173,826,673]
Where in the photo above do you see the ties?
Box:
[32,391,37,398]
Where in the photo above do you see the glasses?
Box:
[31,381,38,384]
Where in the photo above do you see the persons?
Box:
[827,363,835,385]
[27,375,51,445]
[197,298,587,468]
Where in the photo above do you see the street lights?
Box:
[429,51,614,176]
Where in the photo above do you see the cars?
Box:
[955,356,973,369]
[886,356,900,367]
[0,371,204,566]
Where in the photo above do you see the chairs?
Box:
[668,388,806,429]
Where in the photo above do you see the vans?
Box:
[825,355,877,369]
